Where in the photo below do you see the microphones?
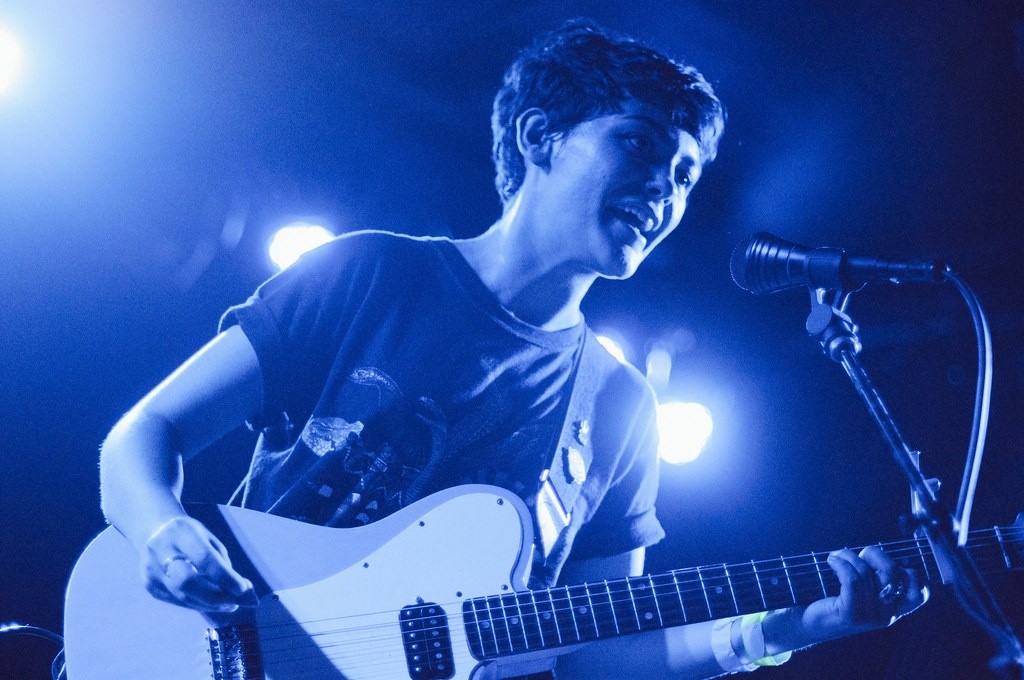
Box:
[730,231,949,296]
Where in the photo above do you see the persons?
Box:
[98,16,932,680]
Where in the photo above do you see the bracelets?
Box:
[703,608,794,673]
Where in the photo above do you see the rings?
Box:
[877,584,902,605]
[159,551,188,577]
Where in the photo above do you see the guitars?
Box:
[63,484,1024,680]
